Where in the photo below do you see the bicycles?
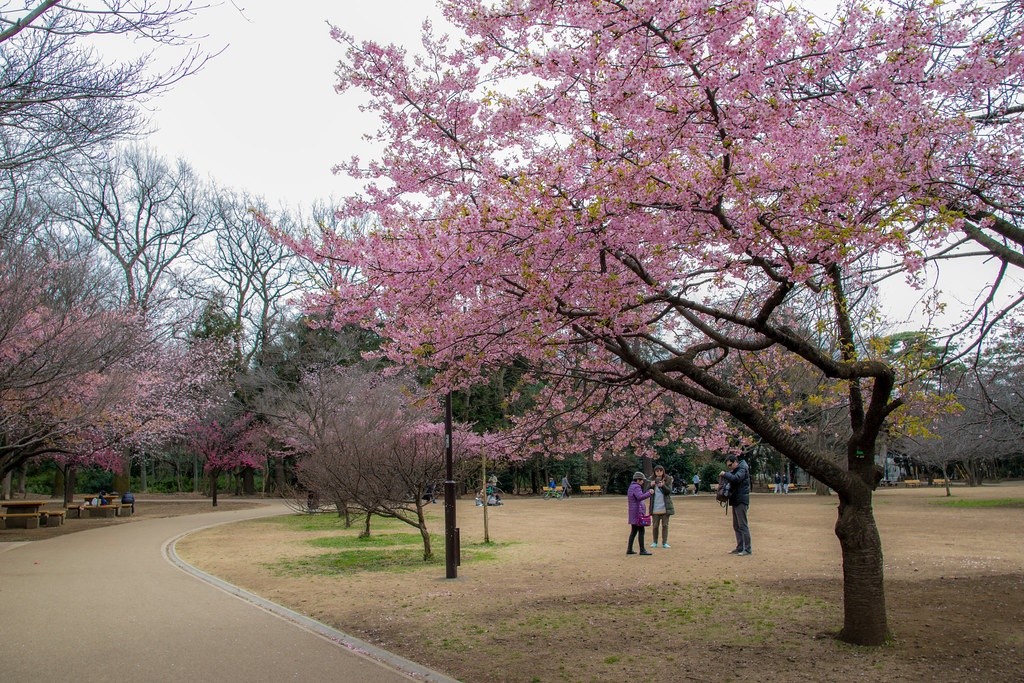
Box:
[543,486,564,501]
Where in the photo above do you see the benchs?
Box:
[795,484,809,492]
[580,485,601,497]
[710,484,720,492]
[541,485,566,497]
[768,483,797,493]
[0,510,66,528]
[933,478,951,487]
[67,503,133,518]
[904,479,921,488]
[686,485,696,494]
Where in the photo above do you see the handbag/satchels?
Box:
[638,514,651,526]
[716,475,734,502]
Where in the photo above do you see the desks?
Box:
[0,501,48,529]
[84,495,119,519]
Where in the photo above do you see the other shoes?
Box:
[568,496,571,498]
[693,494,697,496]
[663,544,671,548]
[627,551,637,554]
[651,544,658,547]
[640,551,652,555]
[433,501,437,504]
[738,550,750,556]
[552,496,555,498]
[728,549,742,554]
[562,497,565,499]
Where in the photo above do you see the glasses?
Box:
[656,470,662,472]
[727,463,733,468]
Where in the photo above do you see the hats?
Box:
[100,490,107,497]
[633,472,647,480]
[654,465,664,470]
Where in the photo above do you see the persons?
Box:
[692,472,701,495]
[719,454,751,556]
[646,465,675,548]
[774,472,789,494]
[122,490,135,514]
[548,478,556,496]
[476,474,504,506]
[560,474,571,499]
[626,471,655,555]
